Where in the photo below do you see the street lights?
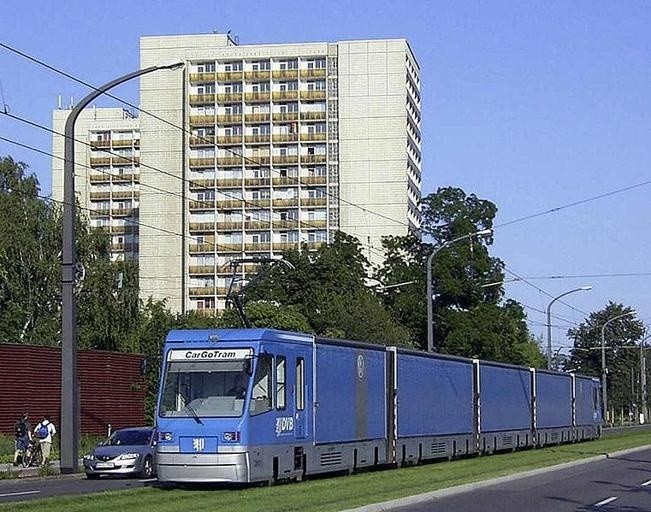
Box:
[427,229,491,353]
[601,311,637,420]
[547,286,592,370]
[60,57,185,474]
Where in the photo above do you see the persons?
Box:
[33,414,57,466]
[13,411,33,465]
[226,373,247,401]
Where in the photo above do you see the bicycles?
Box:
[22,439,42,468]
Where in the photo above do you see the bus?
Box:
[140,329,604,489]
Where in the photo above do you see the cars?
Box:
[83,427,157,479]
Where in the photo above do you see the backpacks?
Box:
[14,419,28,437]
[36,420,51,439]
